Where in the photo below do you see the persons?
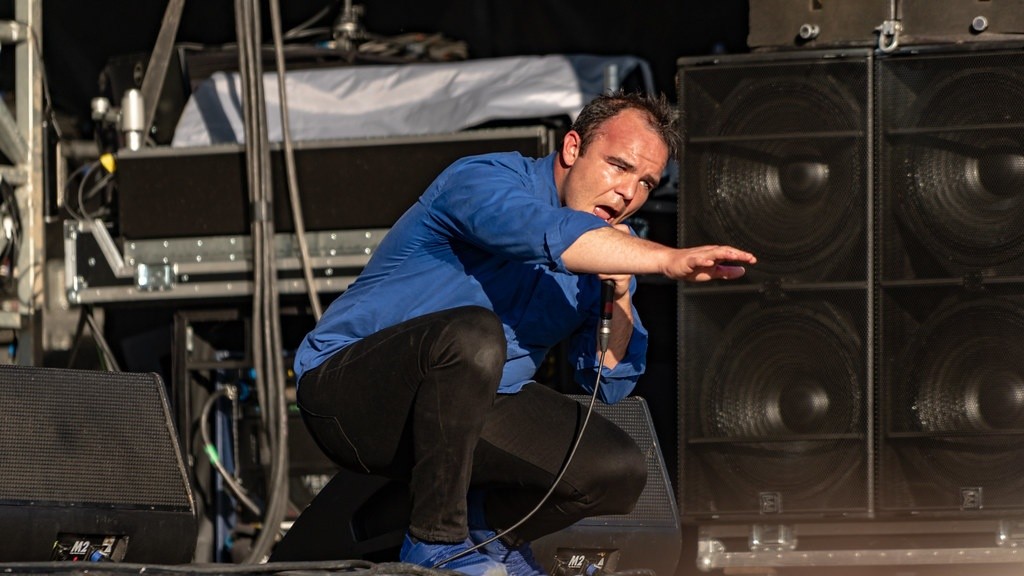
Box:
[294,88,756,576]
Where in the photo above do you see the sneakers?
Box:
[469,529,549,576]
[400,533,507,576]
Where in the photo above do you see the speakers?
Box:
[674,38,1024,527]
[0,365,197,563]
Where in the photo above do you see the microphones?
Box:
[599,279,616,352]
